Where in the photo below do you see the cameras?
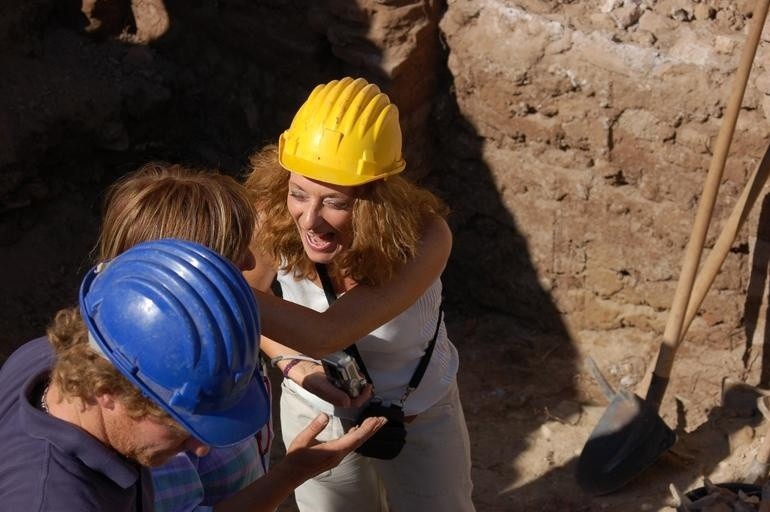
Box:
[320,354,367,398]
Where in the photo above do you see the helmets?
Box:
[77,237,274,450]
[276,73,409,190]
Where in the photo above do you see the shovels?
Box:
[574,0,769,496]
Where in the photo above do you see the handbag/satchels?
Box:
[337,393,408,461]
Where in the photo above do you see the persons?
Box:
[1,237,271,511]
[88,163,387,511]
[235,76,477,511]
